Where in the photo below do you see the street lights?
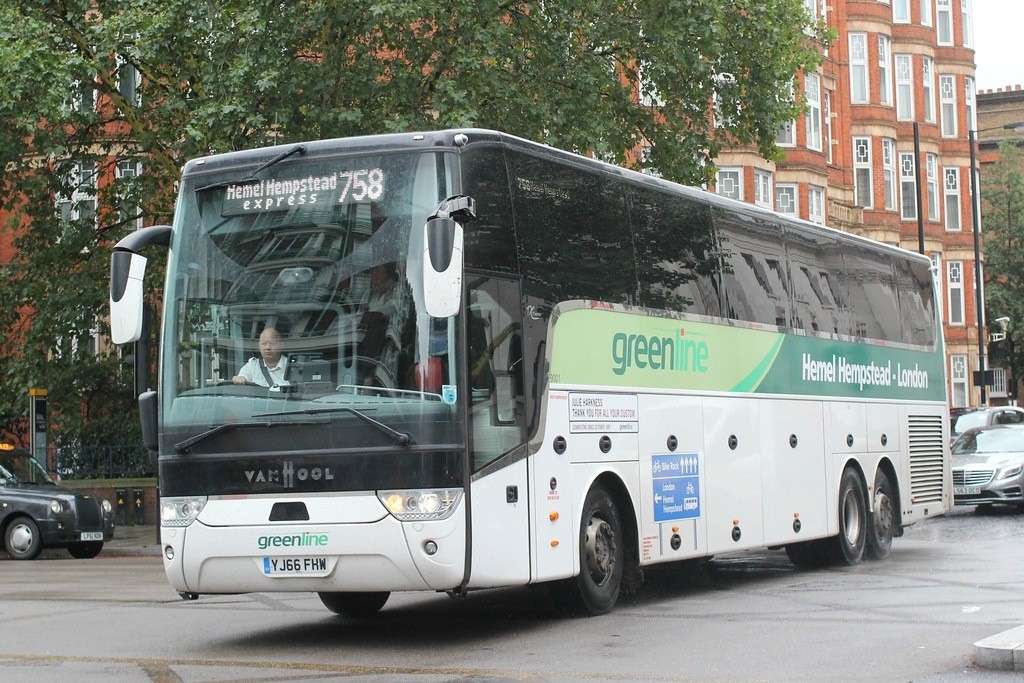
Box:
[967,121,1023,404]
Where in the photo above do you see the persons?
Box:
[232,328,296,392]
[298,253,408,393]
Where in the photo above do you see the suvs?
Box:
[0,443,116,561]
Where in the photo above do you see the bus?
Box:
[103,128,953,620]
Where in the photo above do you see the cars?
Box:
[950,422,1024,510]
[955,405,1024,436]
